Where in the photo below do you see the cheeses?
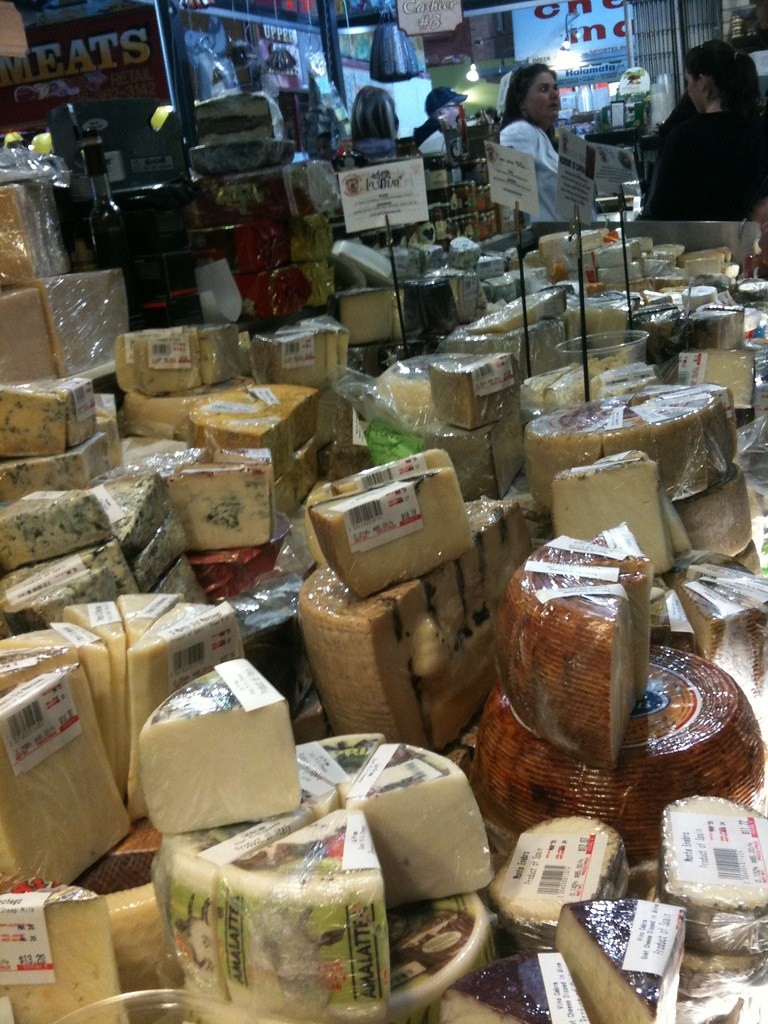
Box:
[2,93,767,1024]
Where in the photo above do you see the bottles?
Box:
[84,144,126,247]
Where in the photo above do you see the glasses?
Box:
[439,103,460,109]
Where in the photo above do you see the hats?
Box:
[425,87,468,116]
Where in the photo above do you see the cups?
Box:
[649,72,674,129]
[51,989,282,1024]
[554,330,651,367]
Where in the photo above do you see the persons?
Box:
[305,129,345,220]
[413,85,465,149]
[636,40,767,266]
[499,63,601,224]
[351,85,419,168]
